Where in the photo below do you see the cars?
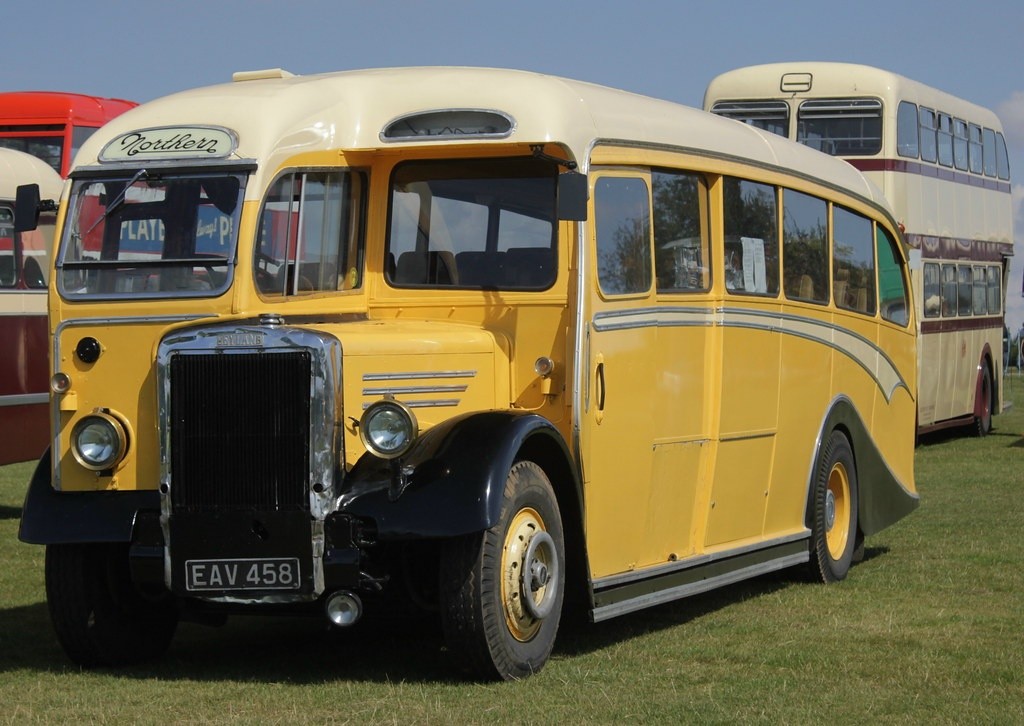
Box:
[0,147,85,469]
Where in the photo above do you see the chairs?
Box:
[394,250,453,284]
[786,252,814,300]
[833,268,851,307]
[278,263,336,291]
[506,247,552,287]
[848,271,867,311]
[455,251,507,284]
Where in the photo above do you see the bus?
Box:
[771,234,872,313]
[701,61,1014,448]
[636,237,785,293]
[1,91,306,279]
[15,63,923,681]
